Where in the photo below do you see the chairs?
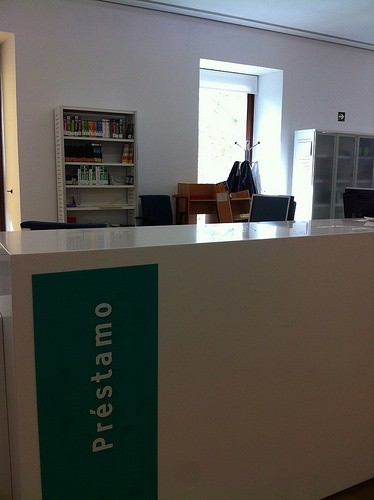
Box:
[136,194,173,226]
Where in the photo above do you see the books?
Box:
[65,165,109,185]
[121,143,134,164]
[63,142,103,164]
[61,115,132,139]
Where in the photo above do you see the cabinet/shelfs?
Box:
[291,129,374,219]
[172,182,250,223]
[55,104,138,228]
